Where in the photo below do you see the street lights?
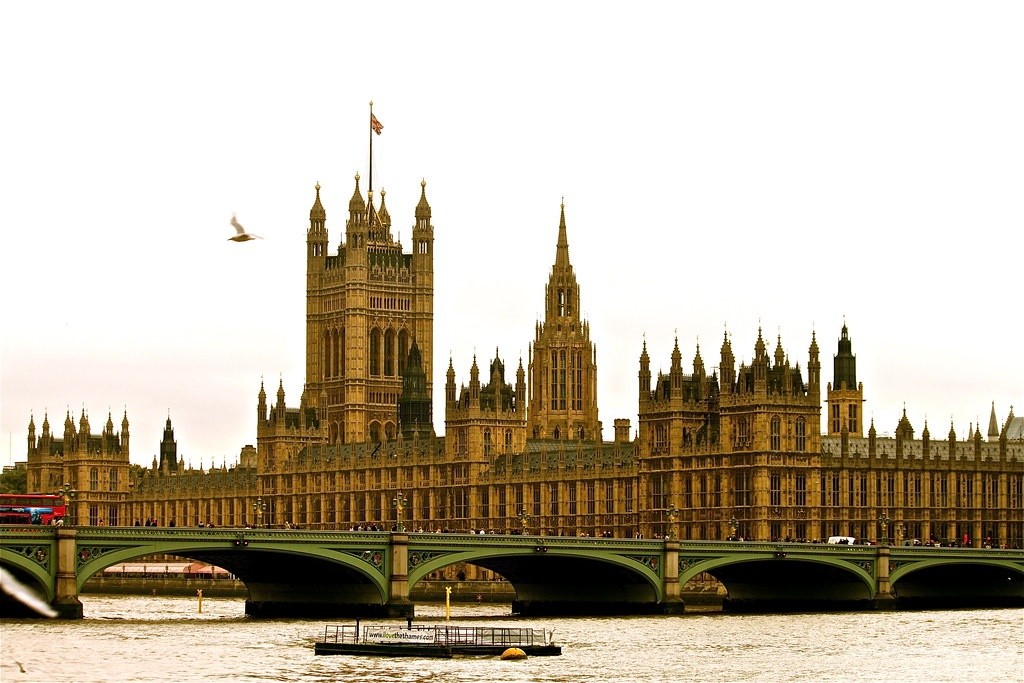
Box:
[728,515,739,538]
[393,492,407,531]
[253,497,266,529]
[879,512,889,547]
[666,503,679,539]
[517,508,530,532]
[58,482,75,525]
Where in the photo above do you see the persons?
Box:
[0,500,1024,556]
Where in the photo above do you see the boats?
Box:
[436,624,563,656]
[315,618,454,658]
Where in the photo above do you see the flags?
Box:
[372,115,383,136]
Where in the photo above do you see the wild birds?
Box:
[226,216,264,242]
[16,661,26,674]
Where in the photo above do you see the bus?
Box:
[0,493,65,524]
